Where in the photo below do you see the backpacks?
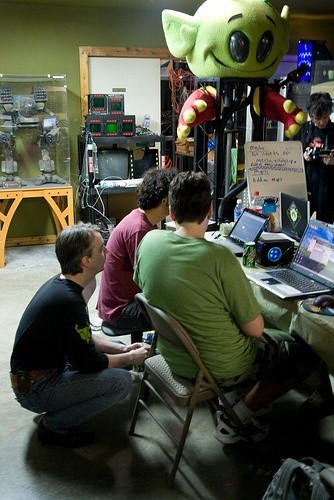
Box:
[263,457,334,500]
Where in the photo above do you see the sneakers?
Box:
[213,384,273,444]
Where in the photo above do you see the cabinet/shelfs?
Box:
[77,134,165,226]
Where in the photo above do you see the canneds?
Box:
[242,241,256,267]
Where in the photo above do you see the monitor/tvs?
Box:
[92,147,159,185]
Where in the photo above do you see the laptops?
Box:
[246,220,334,299]
[279,192,310,240]
[213,208,270,256]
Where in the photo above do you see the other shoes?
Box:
[38,417,93,449]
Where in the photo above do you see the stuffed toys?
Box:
[161,0,305,188]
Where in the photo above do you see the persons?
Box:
[96,168,304,445]
[292,92,334,224]
[11,225,154,461]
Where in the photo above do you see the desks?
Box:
[204,231,334,372]
[0,178,73,268]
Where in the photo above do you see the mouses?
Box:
[314,295,334,308]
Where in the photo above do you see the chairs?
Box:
[128,292,261,489]
